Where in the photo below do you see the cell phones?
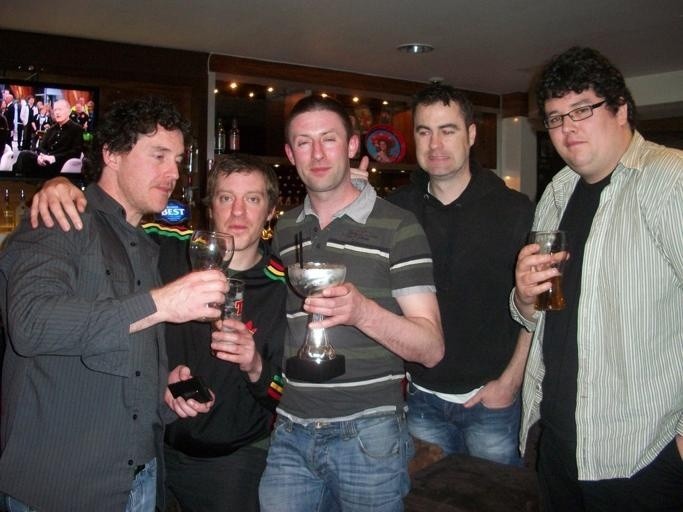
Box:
[168,375,213,403]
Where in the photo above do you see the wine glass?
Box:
[287,261,347,361]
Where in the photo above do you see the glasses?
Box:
[543,99,608,129]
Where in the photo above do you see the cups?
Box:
[187,230,246,359]
[531,230,567,313]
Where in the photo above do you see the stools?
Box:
[403,446,451,476]
[405,449,540,511]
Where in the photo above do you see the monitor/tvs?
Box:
[0,78,100,185]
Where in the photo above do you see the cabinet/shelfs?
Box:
[205,55,503,232]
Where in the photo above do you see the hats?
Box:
[370,133,395,149]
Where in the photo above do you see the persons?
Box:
[0,89,95,173]
[30,152,288,512]
[0,92,230,512]
[509,45,683,512]
[270,95,445,512]
[384,85,536,512]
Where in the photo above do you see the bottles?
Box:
[215,117,240,153]
[0,189,28,231]
